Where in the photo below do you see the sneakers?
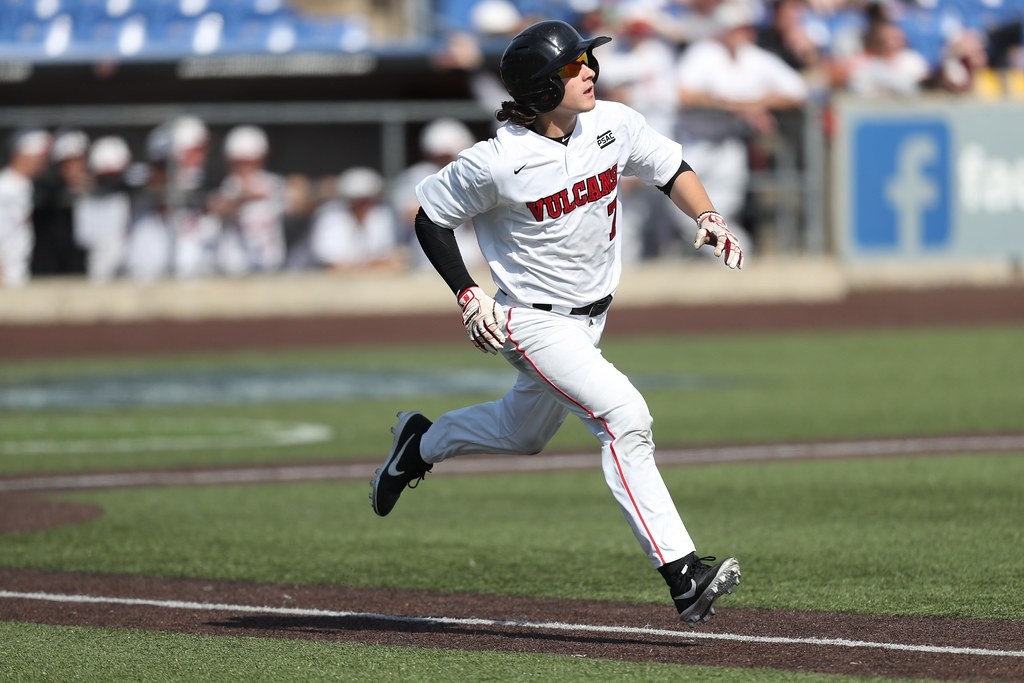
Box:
[670,554,741,627]
[368,410,433,518]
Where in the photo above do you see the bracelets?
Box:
[696,211,721,225]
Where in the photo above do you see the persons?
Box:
[575,0,1024,258]
[366,18,741,625]
[0,117,495,289]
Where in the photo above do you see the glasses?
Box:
[556,50,590,79]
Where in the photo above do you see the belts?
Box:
[499,288,612,317]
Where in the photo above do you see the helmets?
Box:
[499,20,611,113]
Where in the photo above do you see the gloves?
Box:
[456,286,508,356]
[693,211,743,271]
